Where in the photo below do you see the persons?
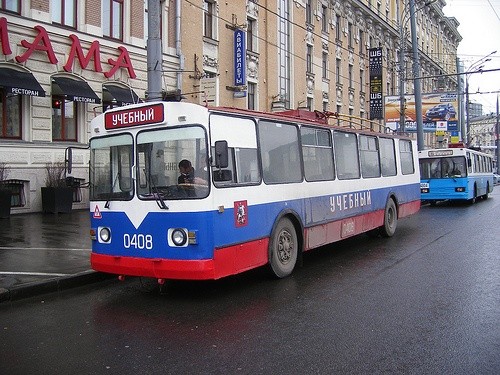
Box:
[452,167,461,175]
[175,159,210,194]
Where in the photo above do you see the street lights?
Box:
[466,51,497,147]
[400,0,439,135]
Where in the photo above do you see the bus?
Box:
[64,90,423,285]
[415,148,493,207]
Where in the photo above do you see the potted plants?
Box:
[0,161,12,220]
[41,160,73,214]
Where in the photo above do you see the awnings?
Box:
[51,75,101,104]
[102,83,144,107]
[0,65,46,98]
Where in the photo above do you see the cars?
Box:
[425,103,456,120]
